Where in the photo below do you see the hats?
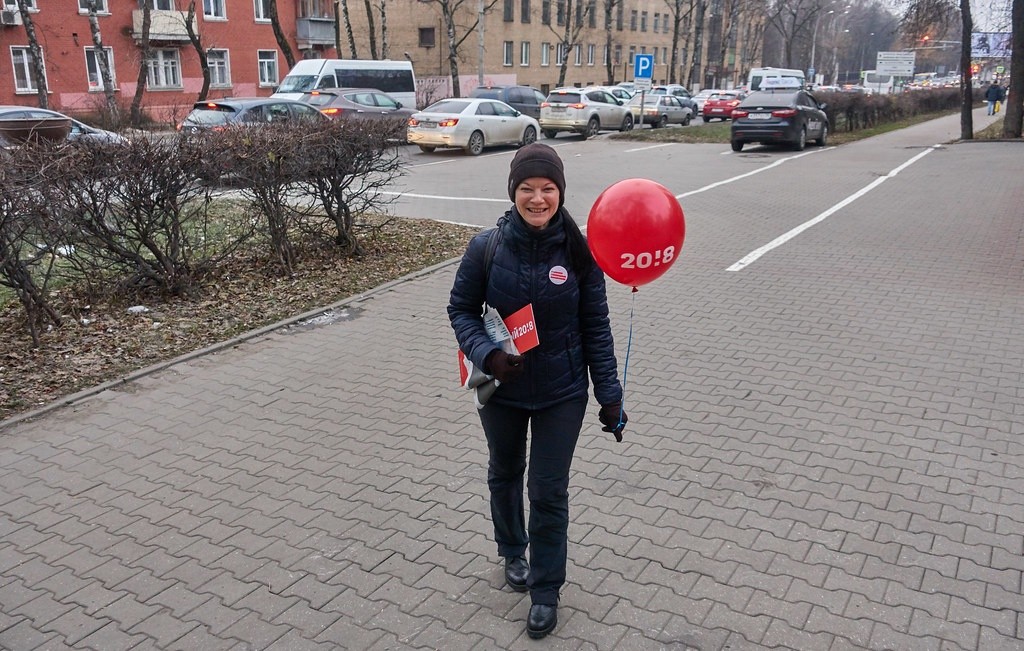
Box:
[507,144,566,208]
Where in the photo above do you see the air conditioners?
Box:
[0,10,22,25]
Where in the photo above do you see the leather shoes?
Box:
[526,600,557,640]
[505,552,531,590]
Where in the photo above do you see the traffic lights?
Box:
[924,35,929,46]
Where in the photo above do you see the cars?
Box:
[593,82,653,104]
[626,94,692,128]
[703,91,748,122]
[911,76,979,89]
[730,77,831,152]
[807,85,872,97]
[0,104,133,181]
[406,98,542,156]
[691,89,719,112]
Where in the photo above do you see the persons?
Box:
[984,79,1007,115]
[447,141,631,636]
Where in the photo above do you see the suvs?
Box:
[539,87,634,139]
[468,86,547,118]
[176,97,371,184]
[298,88,421,145]
[645,84,699,118]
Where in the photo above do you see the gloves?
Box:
[482,349,524,383]
[598,402,628,443]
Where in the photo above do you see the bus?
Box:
[915,73,937,82]
[863,70,909,96]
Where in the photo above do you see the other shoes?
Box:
[988,112,990,115]
[992,112,995,115]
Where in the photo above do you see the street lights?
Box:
[781,4,851,80]
[860,33,874,83]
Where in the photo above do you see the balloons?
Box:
[586,179,685,288]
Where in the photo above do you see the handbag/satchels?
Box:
[994,100,1000,112]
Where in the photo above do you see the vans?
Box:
[746,68,807,94]
[270,59,418,108]
